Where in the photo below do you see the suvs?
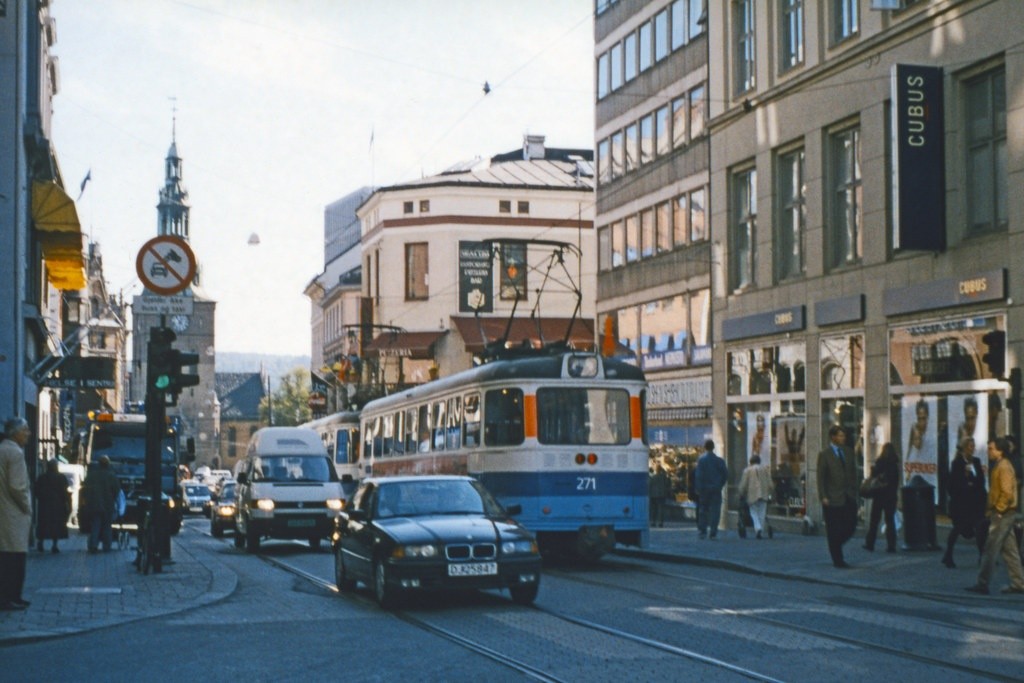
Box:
[210,479,237,536]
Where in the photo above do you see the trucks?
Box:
[79,410,196,534]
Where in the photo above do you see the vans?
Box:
[182,480,212,515]
[210,470,231,484]
[234,426,342,553]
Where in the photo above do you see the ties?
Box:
[837,447,845,468]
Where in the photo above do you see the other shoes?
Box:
[52,545,59,553]
[886,547,895,552]
[834,559,849,569]
[1001,584,1024,595]
[862,543,874,552]
[710,532,717,540]
[756,529,763,539]
[942,552,955,567]
[699,532,707,539]
[37,543,43,551]
[965,583,988,594]
[0,598,29,608]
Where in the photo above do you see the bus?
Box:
[359,350,652,564]
[281,411,359,496]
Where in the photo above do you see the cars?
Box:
[334,476,545,608]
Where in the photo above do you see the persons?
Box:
[862,443,898,553]
[906,400,938,503]
[688,440,728,541]
[966,439,1024,593]
[956,398,984,454]
[817,425,860,567]
[86,455,121,552]
[738,455,775,539]
[784,420,804,475]
[650,468,671,527]
[750,415,770,466]
[35,458,71,552]
[0,417,33,610]
[941,435,998,568]
[1005,436,1024,492]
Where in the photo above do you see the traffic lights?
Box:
[151,347,200,395]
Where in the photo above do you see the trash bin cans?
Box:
[137,491,176,560]
[901,474,942,552]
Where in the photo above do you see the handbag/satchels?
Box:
[859,460,888,497]
